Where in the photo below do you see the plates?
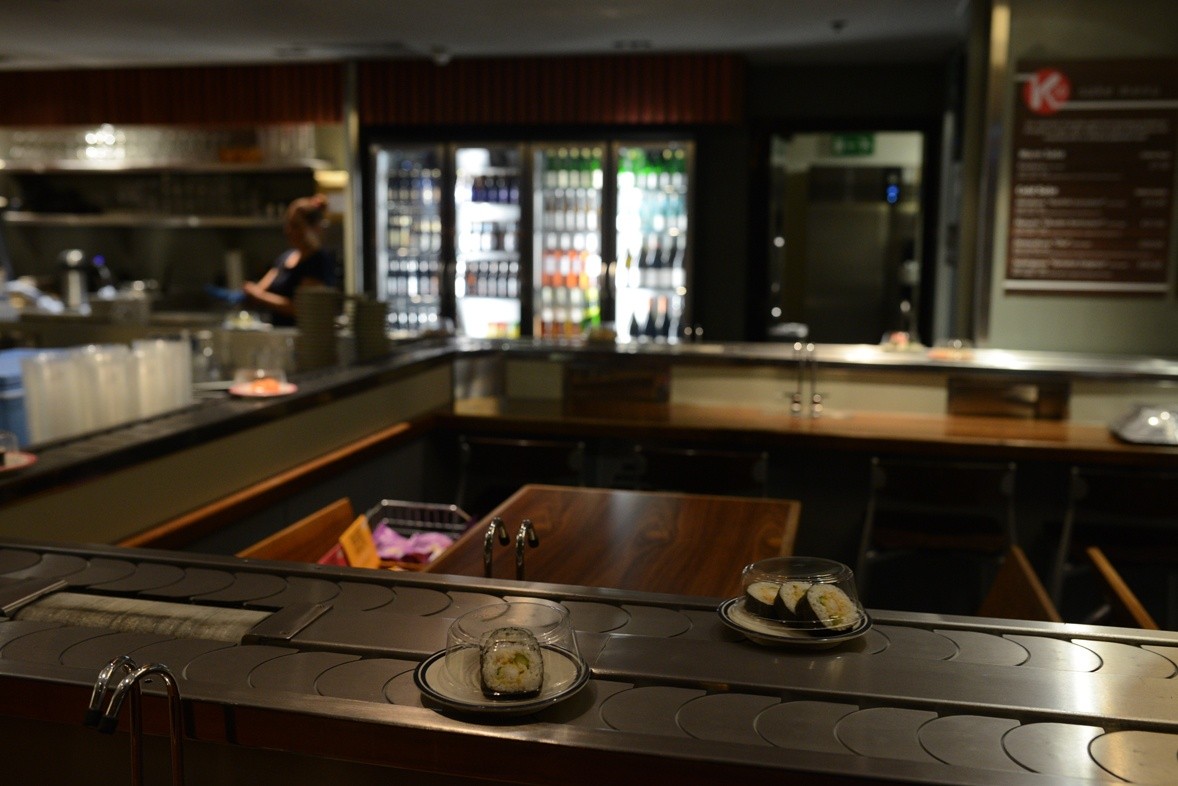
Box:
[717,594,873,652]
[412,644,593,716]
[228,378,297,397]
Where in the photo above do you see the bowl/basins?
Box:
[233,368,289,396]
[1110,401,1178,445]
[879,331,919,351]
[931,336,972,360]
[295,284,395,369]
[438,599,586,702]
[729,554,867,632]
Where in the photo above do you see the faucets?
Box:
[514,519,539,581]
[483,516,510,579]
[782,340,833,420]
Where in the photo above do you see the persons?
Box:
[234,191,343,329]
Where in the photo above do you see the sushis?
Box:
[744,580,859,638]
[479,623,545,700]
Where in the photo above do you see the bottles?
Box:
[465,166,526,297]
[385,166,455,330]
[541,147,601,339]
[616,148,687,339]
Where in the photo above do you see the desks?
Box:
[423,485,801,598]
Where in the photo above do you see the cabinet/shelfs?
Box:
[0,55,356,355]
